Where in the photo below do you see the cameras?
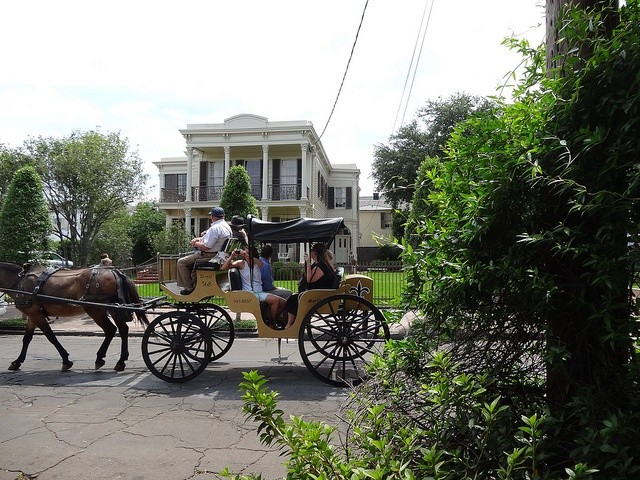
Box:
[234,248,241,255]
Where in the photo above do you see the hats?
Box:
[228,214,246,227]
[208,207,225,217]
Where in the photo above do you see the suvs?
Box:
[18,250,73,268]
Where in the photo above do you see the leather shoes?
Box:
[179,288,194,295]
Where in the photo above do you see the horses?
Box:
[0,261,159,371]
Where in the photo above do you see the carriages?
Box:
[0,213,389,386]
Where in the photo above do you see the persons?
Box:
[325,248,338,277]
[227,215,248,244]
[221,247,286,330]
[259,245,293,319]
[177,206,231,295]
[285,243,338,329]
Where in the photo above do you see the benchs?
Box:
[228,267,269,322]
[297,267,344,301]
[194,237,242,269]
